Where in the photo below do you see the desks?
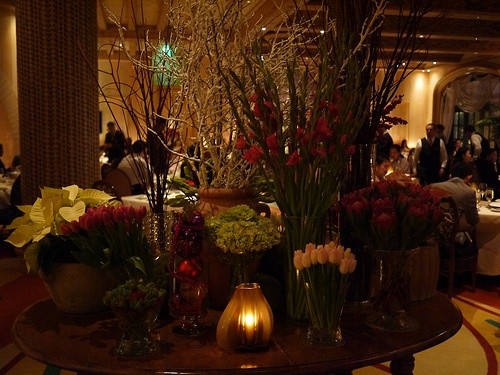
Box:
[457,200,500,278]
[10,291,463,375]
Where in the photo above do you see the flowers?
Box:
[202,204,283,283]
[58,204,155,284]
[216,27,408,321]
[293,241,357,330]
[102,279,168,314]
[335,170,452,253]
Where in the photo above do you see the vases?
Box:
[142,211,180,258]
[303,279,350,347]
[215,283,274,355]
[371,248,418,333]
[195,186,272,301]
[109,304,160,358]
[278,213,339,327]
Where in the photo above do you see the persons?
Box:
[0,144,22,224]
[371,122,500,201]
[99,115,216,195]
[418,161,480,287]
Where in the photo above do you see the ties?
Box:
[430,139,432,145]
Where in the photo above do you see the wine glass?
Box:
[476,192,481,210]
[485,189,494,209]
[478,183,488,204]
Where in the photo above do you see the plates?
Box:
[489,202,500,208]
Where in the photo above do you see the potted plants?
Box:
[5,185,122,315]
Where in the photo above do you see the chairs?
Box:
[424,186,479,298]
